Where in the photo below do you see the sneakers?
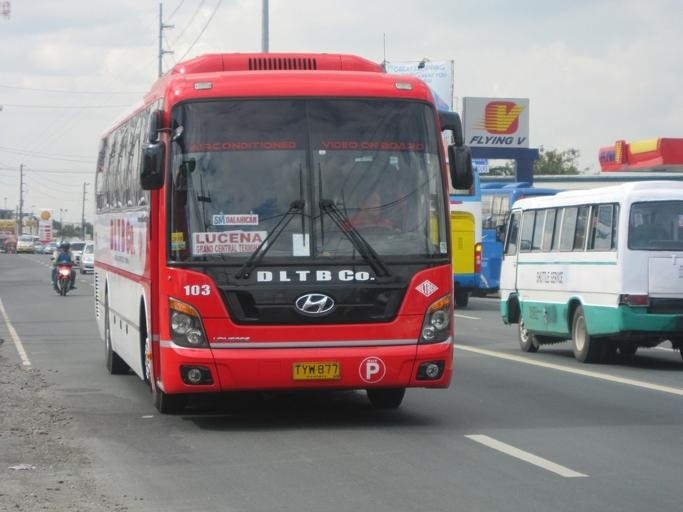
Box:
[70,285,77,289]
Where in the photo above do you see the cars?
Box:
[17,232,95,274]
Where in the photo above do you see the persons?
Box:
[321,150,404,256]
[50,240,78,290]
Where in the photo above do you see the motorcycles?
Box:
[51,261,73,296]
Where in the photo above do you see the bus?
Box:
[94,51,474,414]
[498,179,683,365]
[479,183,556,299]
[448,162,482,308]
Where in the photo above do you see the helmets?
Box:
[57,240,69,250]
